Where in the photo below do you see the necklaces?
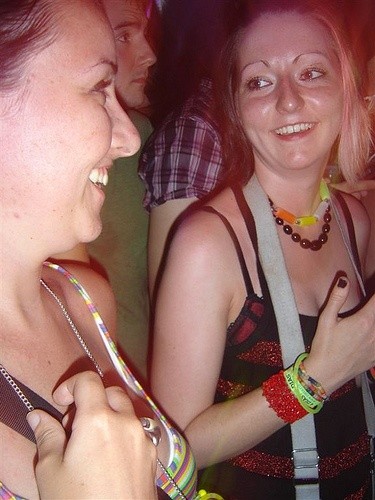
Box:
[0,278,189,500]
[266,195,332,251]
[268,176,330,226]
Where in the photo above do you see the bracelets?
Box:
[261,350,326,424]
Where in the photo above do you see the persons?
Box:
[150,0,375,499]
[53,1,156,377]
[0,1,221,500]
[142,64,235,306]
